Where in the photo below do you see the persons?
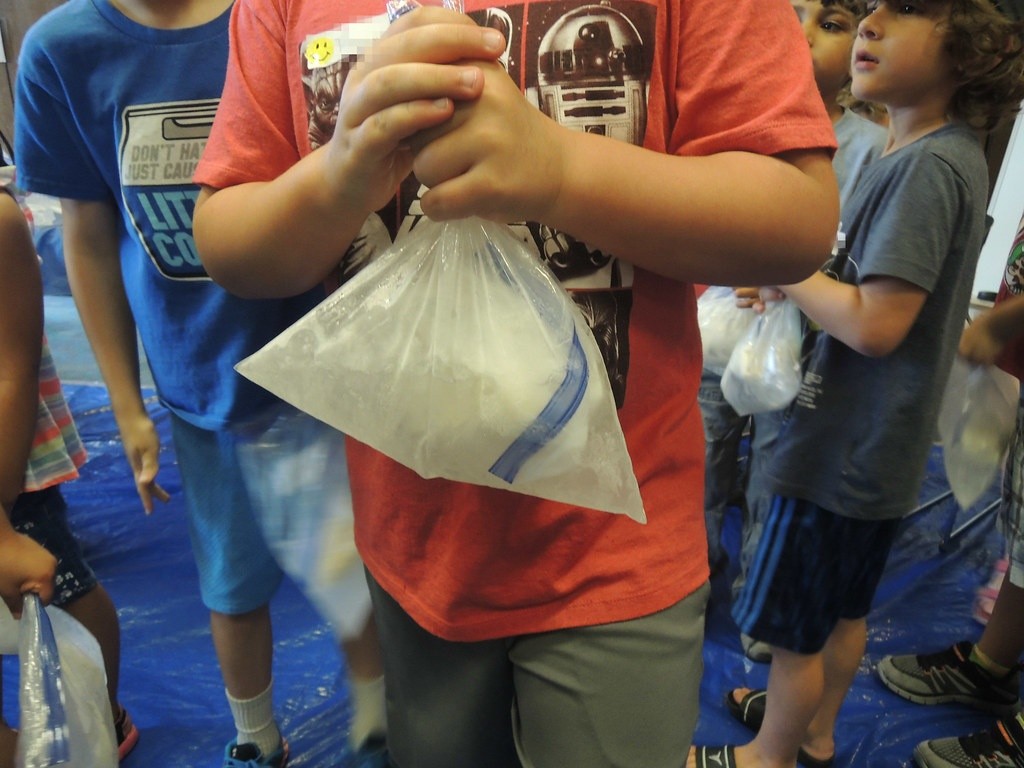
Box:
[0,1,386,767]
[683,1,1024,767]
[192,1,838,768]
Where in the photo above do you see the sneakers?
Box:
[877,640,1022,711]
[913,713,1024,768]
[222,734,288,768]
[114,703,138,760]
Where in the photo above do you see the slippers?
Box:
[696,744,736,768]
[725,688,836,768]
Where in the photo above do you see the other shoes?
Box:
[740,633,773,663]
